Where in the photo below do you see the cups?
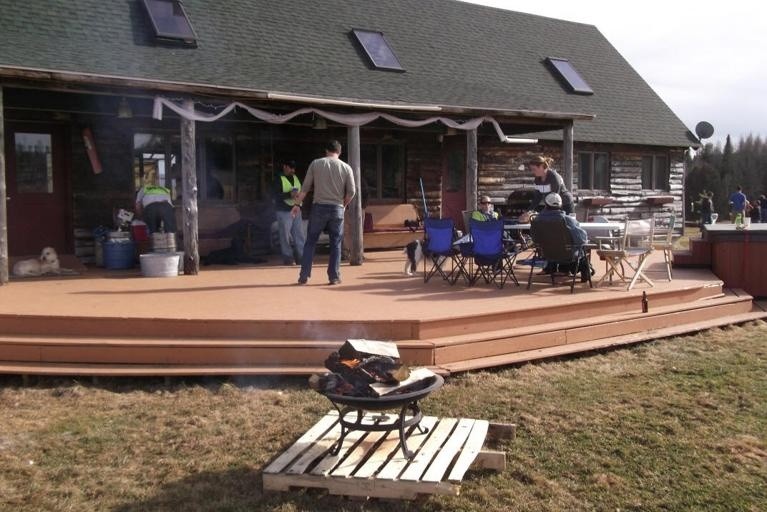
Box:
[743,216,753,228]
[292,186,298,192]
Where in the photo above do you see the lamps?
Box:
[312,112,328,130]
[116,98,133,118]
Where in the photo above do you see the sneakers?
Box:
[536,269,594,283]
[286,259,340,285]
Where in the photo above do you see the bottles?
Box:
[735,211,743,230]
[641,290,649,314]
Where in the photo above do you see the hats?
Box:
[283,159,297,168]
[544,192,562,207]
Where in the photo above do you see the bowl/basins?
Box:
[140,253,183,278]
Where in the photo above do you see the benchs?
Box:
[173,205,243,258]
[340,203,426,251]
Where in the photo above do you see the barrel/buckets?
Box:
[139,250,180,276]
[130,224,148,241]
[106,231,130,243]
[745,216,751,226]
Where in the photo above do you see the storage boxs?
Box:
[129,223,150,241]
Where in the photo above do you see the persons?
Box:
[274,160,305,266]
[290,139,356,285]
[701,185,767,232]
[529,156,576,275]
[529,192,595,282]
[136,184,182,251]
[472,195,502,273]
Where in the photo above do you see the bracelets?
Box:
[295,203,300,207]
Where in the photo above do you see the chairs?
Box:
[421,213,676,295]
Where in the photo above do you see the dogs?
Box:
[202,236,268,266]
[401,227,462,276]
[12,246,73,276]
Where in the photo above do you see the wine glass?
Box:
[710,212,719,230]
